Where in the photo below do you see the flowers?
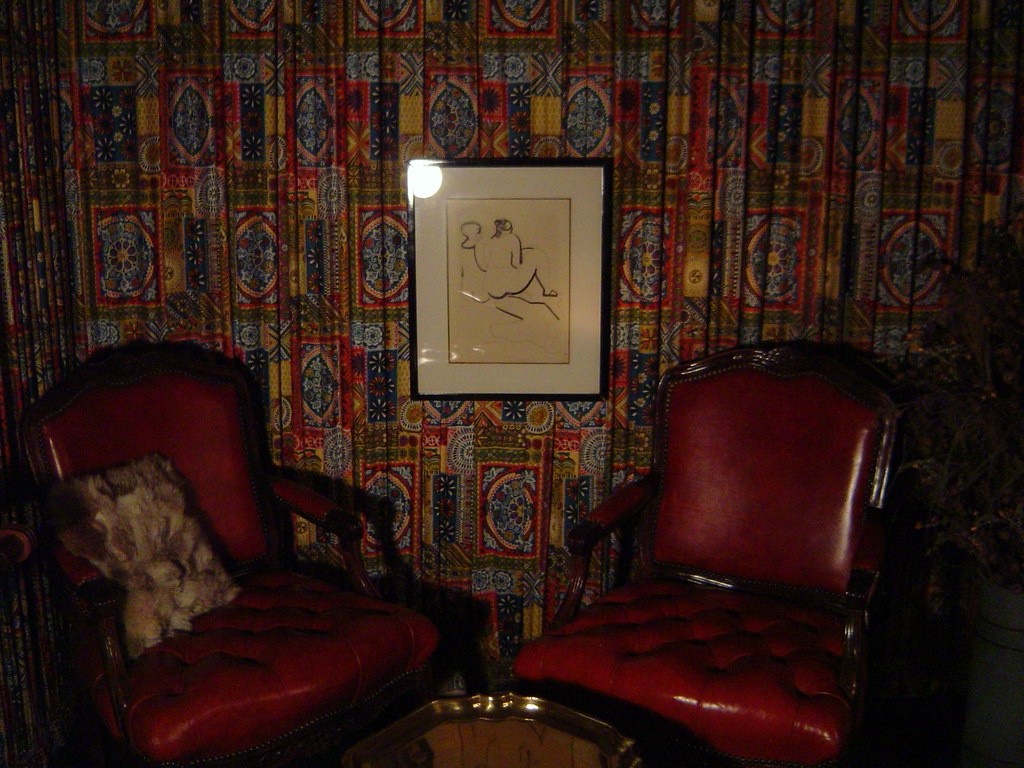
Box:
[885,219,1024,616]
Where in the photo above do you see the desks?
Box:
[340,691,644,768]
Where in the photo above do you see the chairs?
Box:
[0,340,442,768]
[510,338,908,768]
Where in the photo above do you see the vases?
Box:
[957,576,1024,768]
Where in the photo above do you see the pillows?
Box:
[43,450,243,659]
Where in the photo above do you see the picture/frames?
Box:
[406,157,614,403]
